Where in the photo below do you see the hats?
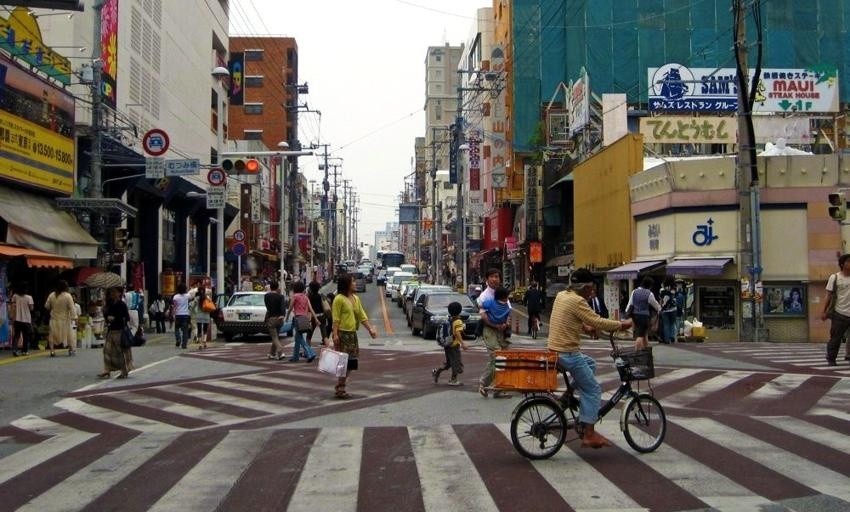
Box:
[571,267,600,284]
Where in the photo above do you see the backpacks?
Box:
[436,316,459,347]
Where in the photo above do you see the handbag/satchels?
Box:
[202,299,216,311]
[292,314,312,333]
[120,324,135,349]
[627,305,634,314]
[825,292,837,315]
[318,348,349,379]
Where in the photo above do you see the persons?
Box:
[331,273,376,399]
[547,268,632,445]
[767,287,804,312]
[432,302,468,386]
[10,275,235,378]
[469,268,514,399]
[819,253,850,364]
[625,275,683,352]
[522,280,544,335]
[261,263,336,363]
[586,289,607,317]
[426,262,457,285]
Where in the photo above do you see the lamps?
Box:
[0,9,104,87]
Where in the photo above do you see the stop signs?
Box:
[232,243,245,255]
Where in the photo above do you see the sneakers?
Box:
[175,340,207,350]
[68,350,77,355]
[827,359,836,365]
[268,346,317,363]
[50,352,55,356]
[11,349,30,358]
[480,383,513,398]
[335,383,353,399]
[448,377,463,386]
[431,367,440,382]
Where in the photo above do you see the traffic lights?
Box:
[112,227,134,264]
[829,192,847,221]
[221,158,261,175]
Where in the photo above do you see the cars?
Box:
[303,266,329,286]
[376,269,387,285]
[333,256,374,292]
[397,280,483,340]
[211,291,295,343]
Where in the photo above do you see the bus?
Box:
[376,250,405,270]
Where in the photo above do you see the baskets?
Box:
[611,346,654,381]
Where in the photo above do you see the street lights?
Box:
[309,179,316,282]
[328,199,333,263]
[433,178,442,284]
[417,197,422,274]
[459,144,470,293]
[277,141,289,296]
[212,66,230,308]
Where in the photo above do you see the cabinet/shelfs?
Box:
[700,288,734,326]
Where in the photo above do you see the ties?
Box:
[594,298,599,312]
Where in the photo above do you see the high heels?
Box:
[97,372,109,378]
[117,374,128,379]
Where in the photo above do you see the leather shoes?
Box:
[583,435,612,448]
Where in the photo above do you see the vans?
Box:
[384,264,419,302]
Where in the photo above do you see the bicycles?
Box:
[511,327,666,459]
[526,303,542,339]
